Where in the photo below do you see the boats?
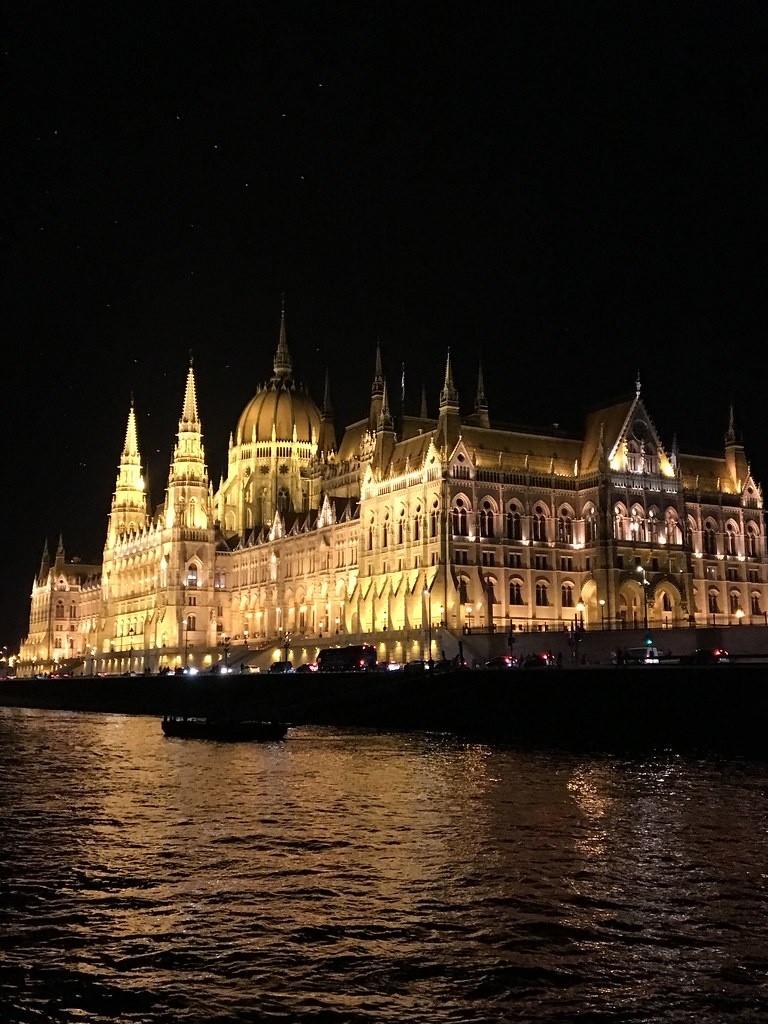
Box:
[161,713,290,742]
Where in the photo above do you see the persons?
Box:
[34,646,672,680]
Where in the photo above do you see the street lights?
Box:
[182,618,188,668]
[128,628,134,677]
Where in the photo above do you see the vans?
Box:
[620,646,660,664]
[267,661,293,673]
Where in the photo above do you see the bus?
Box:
[315,644,378,673]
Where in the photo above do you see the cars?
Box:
[403,660,432,673]
[487,655,520,668]
[523,652,560,668]
[205,664,233,674]
[377,660,401,672]
[235,664,260,674]
[691,647,730,665]
[296,662,319,673]
